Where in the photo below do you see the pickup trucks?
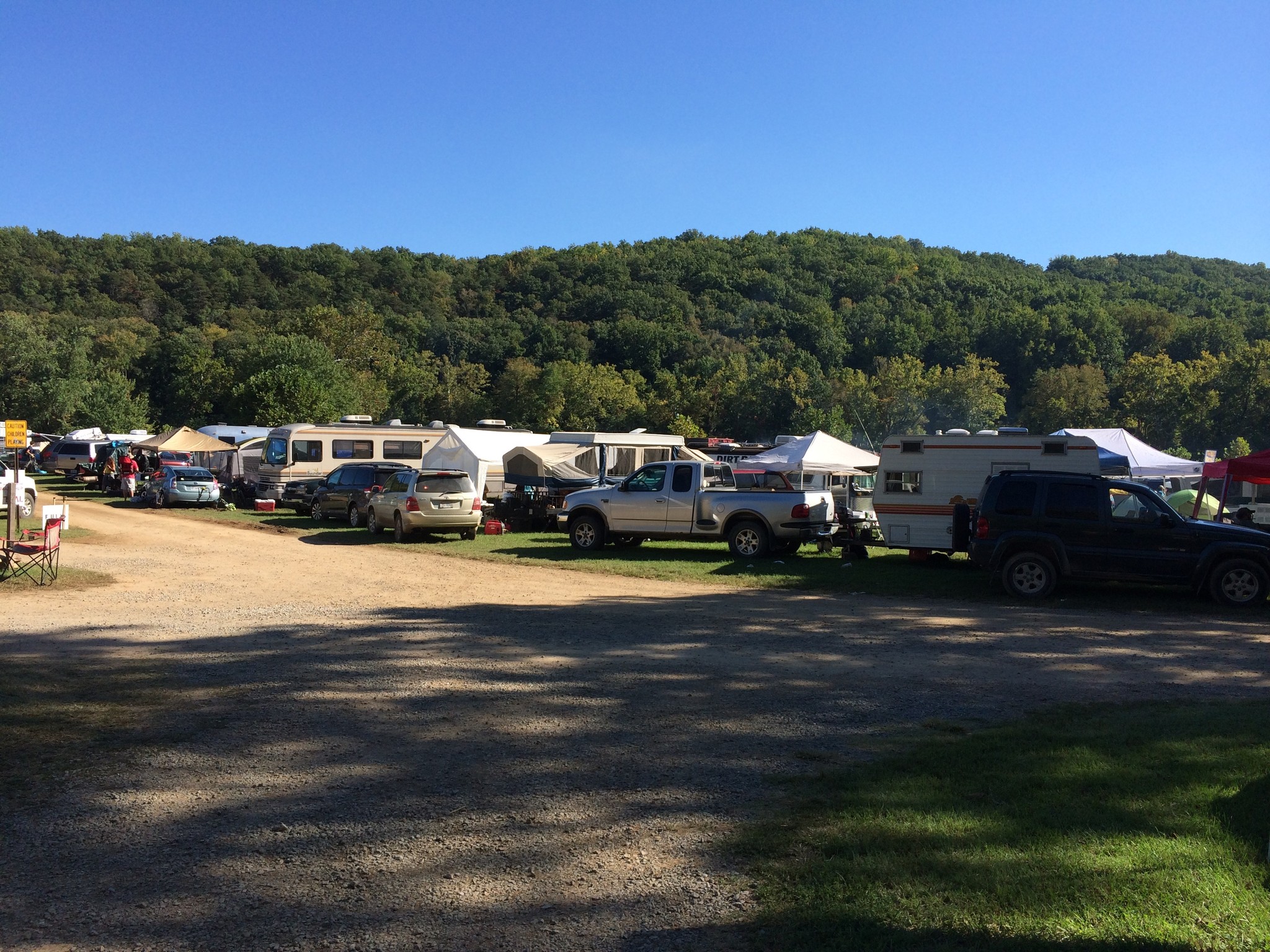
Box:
[556,460,840,560]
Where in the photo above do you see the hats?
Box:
[126,452,135,460]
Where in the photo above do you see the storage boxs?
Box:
[255,499,275,511]
[485,520,502,535]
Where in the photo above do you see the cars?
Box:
[0,421,220,519]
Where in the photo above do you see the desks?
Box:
[867,519,888,549]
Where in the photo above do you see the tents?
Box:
[131,425,240,493]
[1164,489,1230,518]
[1191,447,1270,520]
[1052,428,1213,522]
[736,430,881,491]
[422,428,551,510]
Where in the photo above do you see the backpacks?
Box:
[119,460,134,475]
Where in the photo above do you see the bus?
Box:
[255,414,534,502]
[196,422,276,446]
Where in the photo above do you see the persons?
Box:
[1157,485,1165,497]
[1236,507,1261,531]
[95,458,105,490]
[26,441,35,461]
[119,453,139,502]
[482,483,489,501]
[102,456,117,494]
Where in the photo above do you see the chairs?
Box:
[0,515,66,586]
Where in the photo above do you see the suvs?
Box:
[367,468,483,543]
[952,469,1270,613]
[309,463,413,528]
[281,462,406,517]
